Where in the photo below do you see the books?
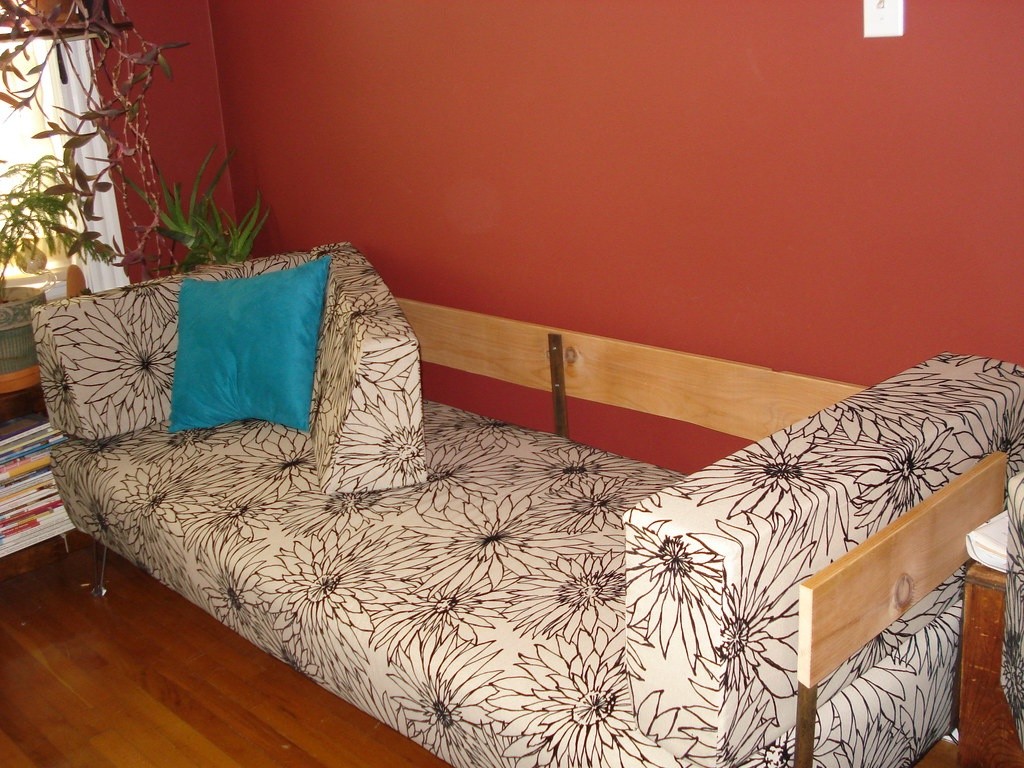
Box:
[0,414,76,559]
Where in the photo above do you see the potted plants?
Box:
[0,153,112,374]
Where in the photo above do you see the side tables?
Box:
[0,365,78,553]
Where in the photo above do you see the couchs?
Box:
[31,248,1024,767]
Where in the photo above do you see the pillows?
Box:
[168,253,332,435]
